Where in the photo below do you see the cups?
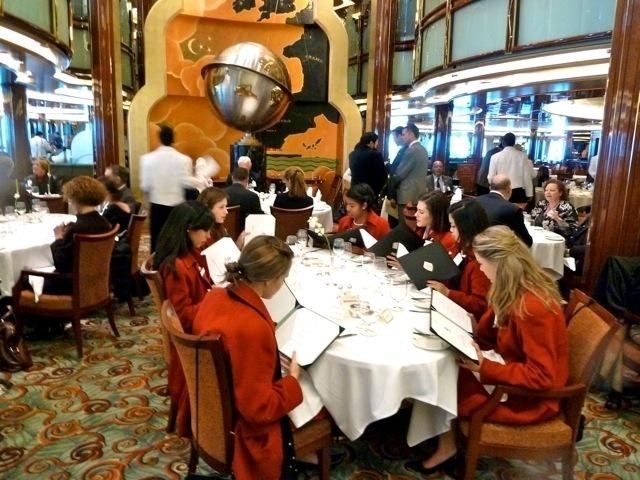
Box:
[333,237,352,259]
[286,228,307,250]
[4,199,48,223]
[362,252,388,277]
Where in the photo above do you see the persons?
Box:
[1,155,139,336]
[427,161,454,192]
[350,122,429,210]
[30,131,72,162]
[139,126,197,256]
[473,132,598,247]
[333,183,571,474]
[152,156,349,480]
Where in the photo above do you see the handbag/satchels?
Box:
[1,304,34,368]
[576,417,586,442]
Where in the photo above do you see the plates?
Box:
[411,339,452,351]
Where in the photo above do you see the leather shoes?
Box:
[407,450,461,474]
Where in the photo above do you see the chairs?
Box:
[141,168,597,434]
[158,296,331,480]
[0,191,151,368]
[448,301,620,480]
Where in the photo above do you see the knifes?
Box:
[412,298,432,300]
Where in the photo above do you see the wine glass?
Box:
[389,277,408,312]
